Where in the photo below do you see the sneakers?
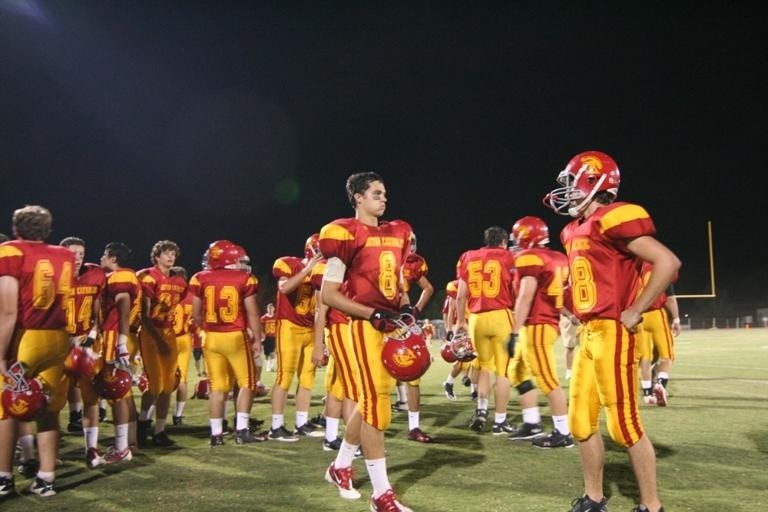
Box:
[506,424,542,440]
[444,376,478,402]
[138,411,183,445]
[210,418,265,448]
[27,476,58,497]
[532,430,576,449]
[469,409,487,433]
[268,426,300,443]
[370,490,415,512]
[0,474,16,503]
[567,494,608,511]
[84,443,137,467]
[310,415,324,428]
[492,421,519,435]
[68,407,108,433]
[391,400,410,413]
[322,438,363,457]
[643,382,670,408]
[14,443,63,478]
[295,425,322,437]
[324,459,362,499]
[407,428,432,443]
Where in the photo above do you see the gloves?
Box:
[445,330,454,341]
[84,337,102,359]
[368,308,399,333]
[117,343,130,367]
[399,305,421,326]
[507,331,519,358]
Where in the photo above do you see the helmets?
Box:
[64,343,98,381]
[409,229,417,256]
[543,149,621,218]
[201,240,254,272]
[94,365,133,399]
[138,366,179,397]
[383,329,431,384]
[509,213,551,250]
[1,360,51,419]
[439,337,476,365]
[303,232,323,261]
